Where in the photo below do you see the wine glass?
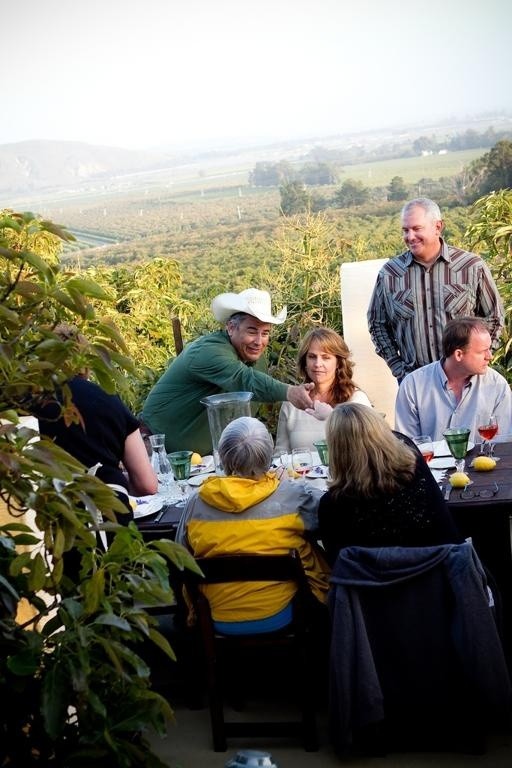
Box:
[165,450,195,510]
[442,427,471,476]
[476,413,500,462]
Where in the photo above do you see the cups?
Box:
[200,391,254,477]
[292,447,314,476]
[412,435,434,463]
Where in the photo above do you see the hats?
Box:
[211,288,288,325]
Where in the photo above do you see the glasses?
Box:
[459,480,500,497]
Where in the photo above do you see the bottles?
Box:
[148,433,175,495]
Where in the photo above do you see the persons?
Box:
[393,315,512,447]
[2,325,162,675]
[137,286,317,458]
[167,412,331,713]
[274,327,374,457]
[366,198,508,387]
[305,398,465,568]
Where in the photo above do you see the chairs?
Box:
[331,540,489,754]
[97,541,208,710]
[184,545,329,755]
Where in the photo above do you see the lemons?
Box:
[473,456,496,471]
[449,472,470,488]
[191,453,202,465]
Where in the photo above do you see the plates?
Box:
[281,452,331,478]
[128,496,163,518]
[186,455,217,487]
[416,439,475,469]
